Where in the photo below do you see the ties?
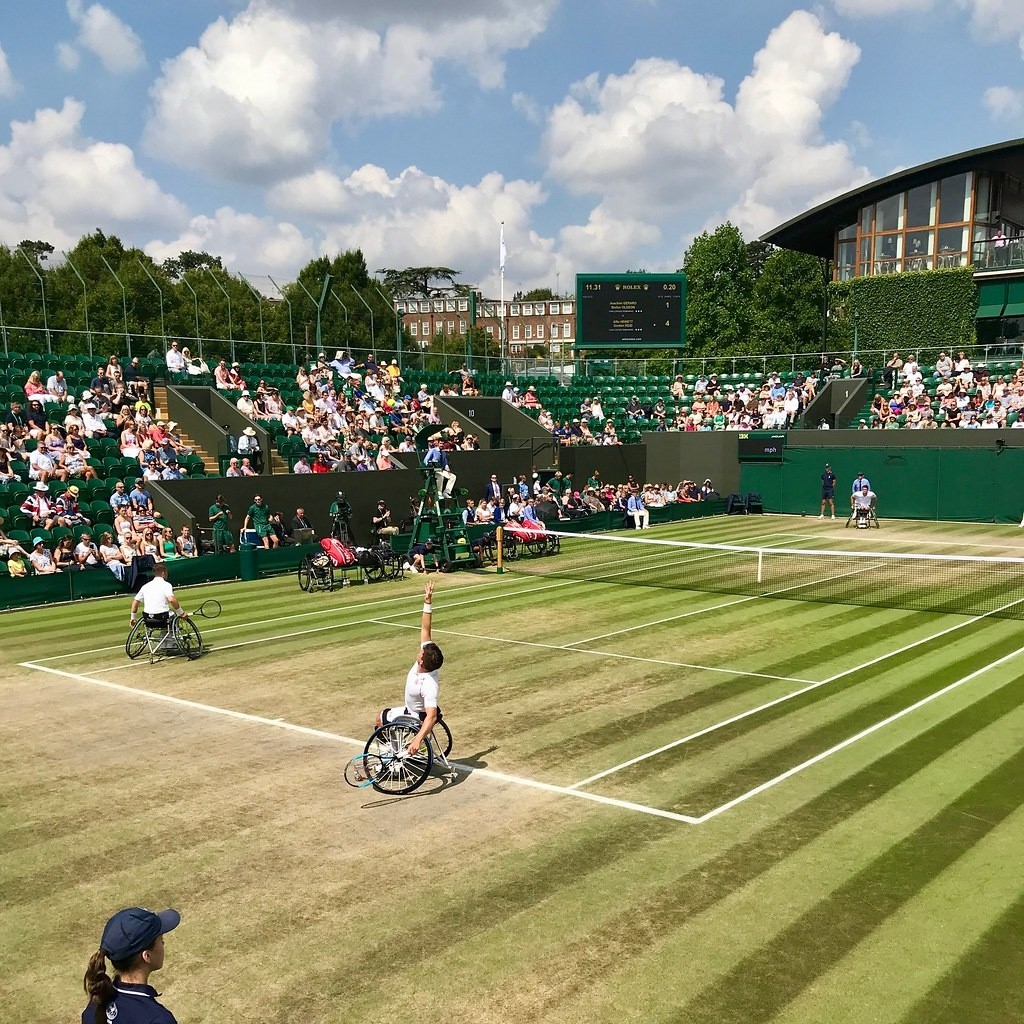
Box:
[301,520,306,528]
[440,450,443,468]
[532,507,535,520]
[494,483,499,498]
[635,497,638,511]
[859,480,862,491]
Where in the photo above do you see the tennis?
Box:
[387,398,396,407]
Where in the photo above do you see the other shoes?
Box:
[437,495,444,499]
[443,492,452,499]
[831,515,835,519]
[817,515,824,520]
[410,566,418,573]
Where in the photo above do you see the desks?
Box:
[941,248,955,267]
[909,251,925,271]
[878,254,892,274]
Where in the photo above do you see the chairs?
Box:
[930,401,940,413]
[896,378,904,389]
[922,377,935,389]
[928,366,936,377]
[978,413,986,422]
[926,389,936,400]
[920,365,930,377]
[897,414,907,427]
[888,390,895,397]
[934,414,945,426]
[966,388,977,400]
[0,345,884,587]
[935,378,943,388]
[971,361,1023,383]
[1005,412,1019,425]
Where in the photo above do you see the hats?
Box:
[0,343,1023,557]
[99,906,181,961]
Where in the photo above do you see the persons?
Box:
[852,471,871,519]
[818,347,1024,430]
[850,485,877,524]
[0,338,817,578]
[993,230,1009,267]
[130,564,189,649]
[81,907,182,1024]
[817,462,837,520]
[375,579,445,779]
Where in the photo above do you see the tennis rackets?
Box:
[343,749,409,788]
[187,599,222,619]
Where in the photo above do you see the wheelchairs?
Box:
[846,506,880,529]
[486,531,518,561]
[125,611,203,665]
[297,552,334,592]
[527,527,560,556]
[363,548,401,580]
[354,707,453,795]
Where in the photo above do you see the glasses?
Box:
[138,510,145,512]
[126,537,133,540]
[145,533,153,536]
[492,477,498,479]
[243,395,249,397]
[40,447,47,449]
[358,423,363,425]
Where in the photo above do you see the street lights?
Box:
[398,309,406,372]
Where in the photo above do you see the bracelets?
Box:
[176,606,185,614]
[131,612,137,621]
[422,602,433,614]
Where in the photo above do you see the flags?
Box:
[498,226,508,272]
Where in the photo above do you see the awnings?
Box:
[975,275,1024,319]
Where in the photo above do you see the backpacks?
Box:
[321,537,373,567]
